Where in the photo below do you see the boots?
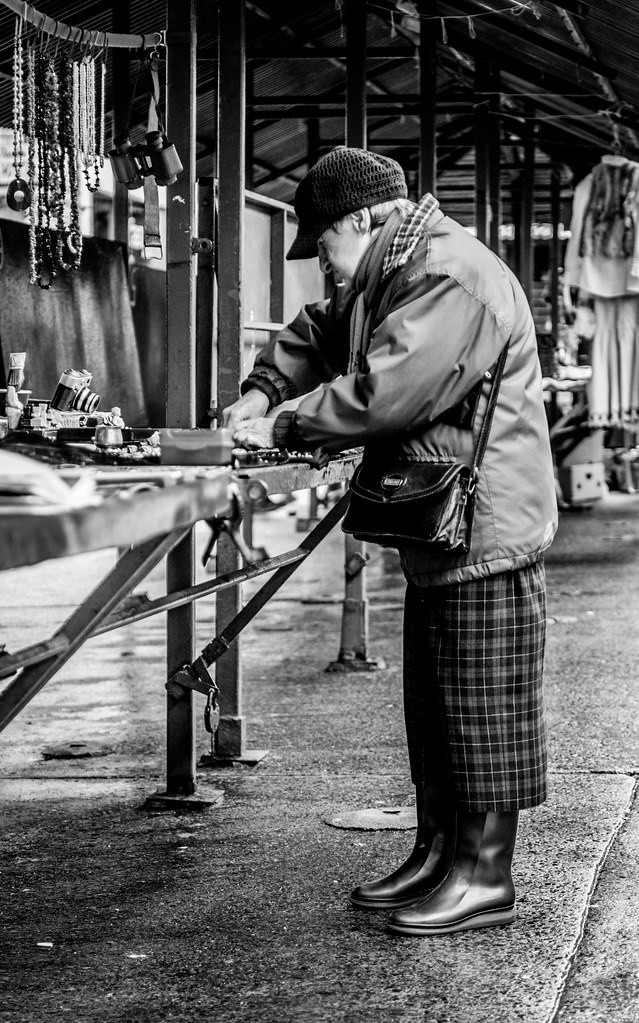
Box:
[388,810,517,936]
[348,805,461,909]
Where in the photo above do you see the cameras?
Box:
[50,368,101,414]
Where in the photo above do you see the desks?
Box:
[0,443,366,808]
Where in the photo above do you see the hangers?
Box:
[609,140,620,158]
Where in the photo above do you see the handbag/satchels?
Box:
[342,457,480,556]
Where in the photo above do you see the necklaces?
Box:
[7,39,106,289]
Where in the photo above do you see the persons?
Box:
[221,145,559,934]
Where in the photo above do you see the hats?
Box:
[286,143,409,261]
